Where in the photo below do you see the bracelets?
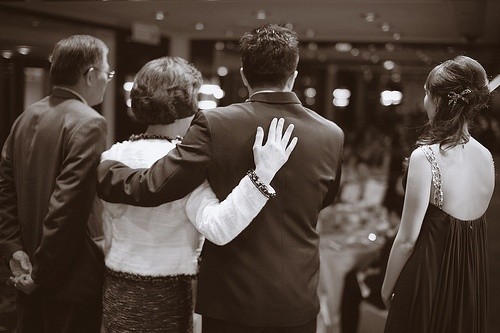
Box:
[246,169,277,200]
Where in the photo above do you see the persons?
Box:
[97,23,344,333]
[0,35,108,333]
[380,56,495,333]
[100,56,297,333]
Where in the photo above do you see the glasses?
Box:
[84,66,115,81]
